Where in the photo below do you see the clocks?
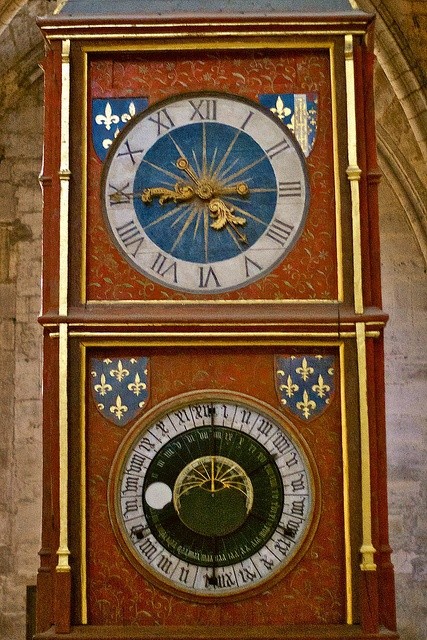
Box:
[38,15,389,320]
[34,320,400,640]
